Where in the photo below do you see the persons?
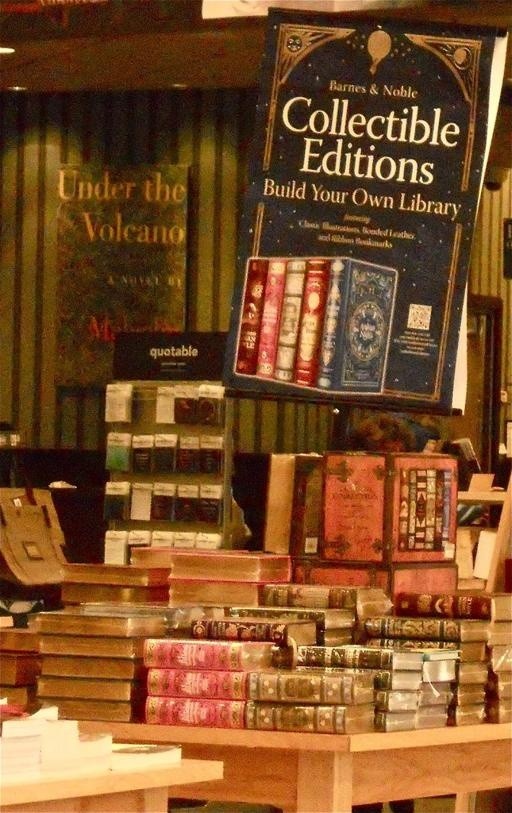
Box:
[341,411,433,812]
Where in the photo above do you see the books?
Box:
[234,252,400,397]
[1,438,511,787]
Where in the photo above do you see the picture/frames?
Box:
[328,290,503,525]
